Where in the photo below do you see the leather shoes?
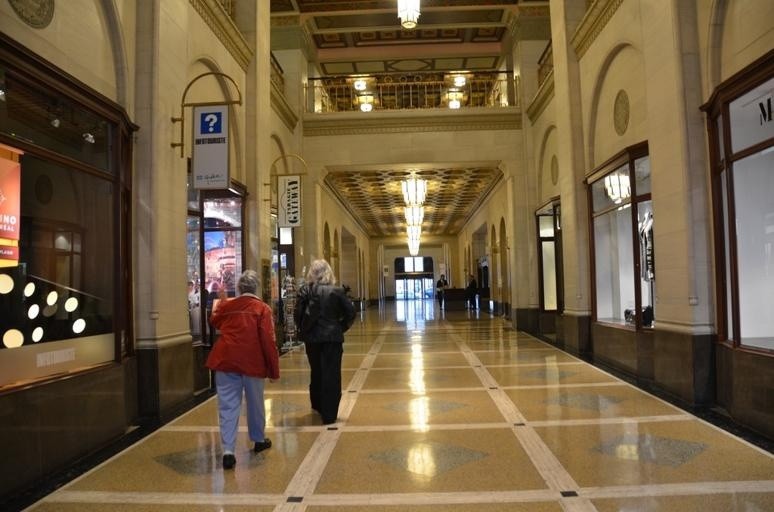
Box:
[254,438,271,452]
[224,454,236,471]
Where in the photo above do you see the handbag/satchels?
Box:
[301,298,319,329]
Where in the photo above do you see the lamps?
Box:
[441,71,473,109]
[345,73,379,112]
[605,171,632,204]
[397,0,421,29]
[401,178,427,256]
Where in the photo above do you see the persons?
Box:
[437,274,448,310]
[206,269,279,470]
[467,275,477,309]
[640,211,656,280]
[189,269,234,337]
[293,258,356,424]
